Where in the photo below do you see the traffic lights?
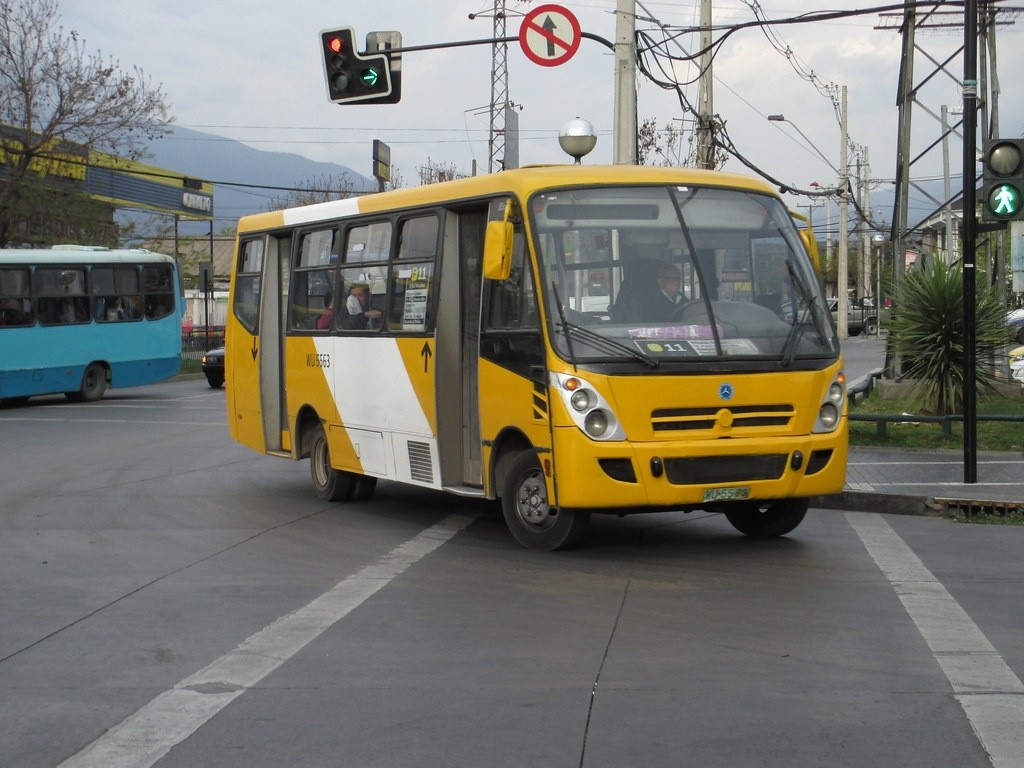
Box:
[201,346,225,389]
[982,138,1024,222]
[320,27,392,103]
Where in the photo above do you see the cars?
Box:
[1008,346,1024,391]
[1005,306,1024,345]
[779,301,802,315]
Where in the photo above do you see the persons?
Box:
[640,263,690,322]
[305,283,382,330]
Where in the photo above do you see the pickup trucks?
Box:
[786,298,891,335]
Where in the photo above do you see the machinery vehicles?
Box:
[224,166,848,553]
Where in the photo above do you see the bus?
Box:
[0,244,186,410]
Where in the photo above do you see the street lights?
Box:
[768,114,849,341]
[871,235,882,339]
